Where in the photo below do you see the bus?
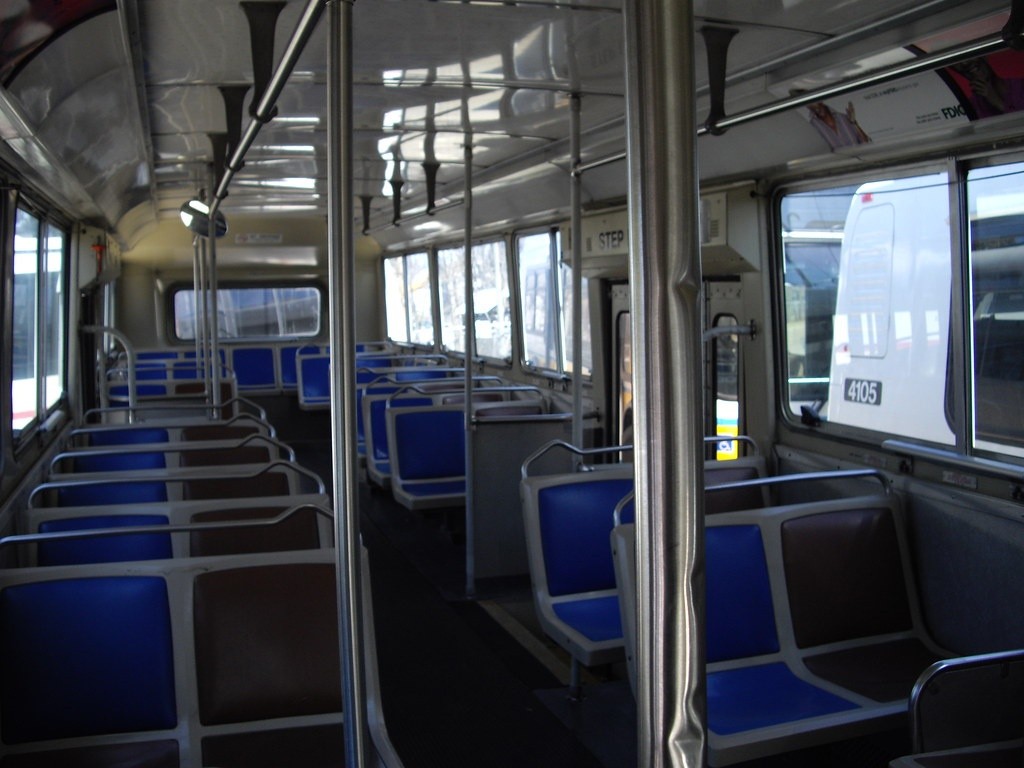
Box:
[524,185,861,421]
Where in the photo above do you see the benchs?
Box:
[0,338,547,768]
[610,466,950,768]
[521,435,770,669]
[889,649,1024,768]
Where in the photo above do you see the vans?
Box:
[827,162,1024,458]
